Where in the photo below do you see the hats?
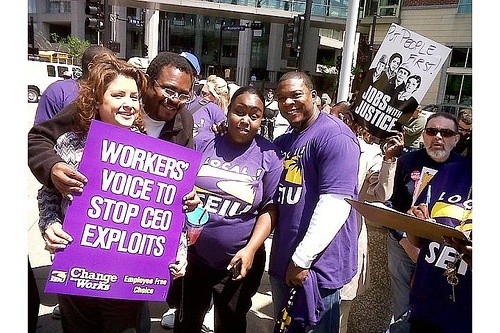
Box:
[456,109,472,129]
[208,77,228,98]
[379,54,388,65]
[398,63,412,75]
[180,52,201,76]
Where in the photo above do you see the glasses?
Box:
[425,128,458,138]
[458,125,470,132]
[201,91,209,97]
[151,77,191,104]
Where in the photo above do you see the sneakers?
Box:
[161,308,177,327]
[386,309,411,333]
[52,302,61,318]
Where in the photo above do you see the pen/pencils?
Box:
[426,185,431,219]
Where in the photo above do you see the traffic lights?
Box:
[142,44,148,57]
[86,2,102,29]
[335,56,342,70]
[358,72,365,79]
[213,49,219,64]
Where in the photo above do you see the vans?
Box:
[28,61,83,103]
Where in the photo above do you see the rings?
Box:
[70,168,77,176]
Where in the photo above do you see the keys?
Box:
[443,252,461,302]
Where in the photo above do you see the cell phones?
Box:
[227,259,241,279]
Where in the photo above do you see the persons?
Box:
[406,160,472,332]
[28,50,202,319]
[172,85,283,333]
[33,46,115,124]
[329,100,404,333]
[36,53,188,333]
[383,111,471,332]
[173,53,472,159]
[274,71,358,333]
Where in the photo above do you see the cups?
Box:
[184,206,209,245]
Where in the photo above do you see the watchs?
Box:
[292,260,308,269]
